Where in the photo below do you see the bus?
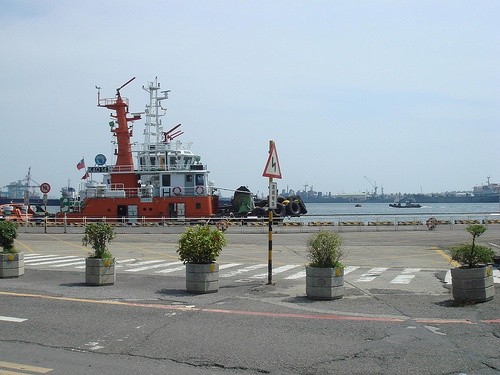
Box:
[455,193,474,197]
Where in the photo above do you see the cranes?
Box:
[362,175,386,197]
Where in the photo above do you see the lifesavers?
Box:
[215,220,228,231]
[172,186,182,196]
[254,207,266,216]
[195,186,204,195]
[295,196,308,215]
[288,201,300,215]
[272,202,285,216]
[228,219,239,225]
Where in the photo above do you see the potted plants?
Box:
[0,219,25,278]
[81,221,117,286]
[438,222,497,305]
[301,228,346,299]
[175,216,231,293]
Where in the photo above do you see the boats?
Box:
[0,75,307,224]
[355,204,362,207]
[389,194,423,208]
